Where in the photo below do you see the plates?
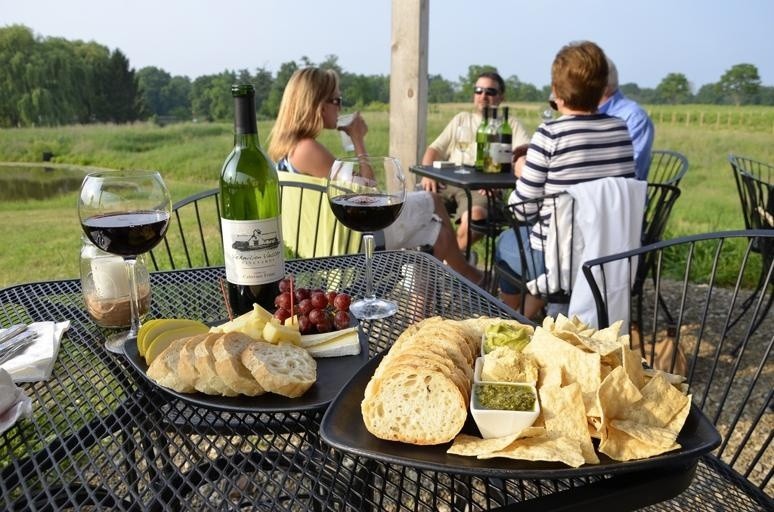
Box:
[122,304,370,415]
[317,344,722,478]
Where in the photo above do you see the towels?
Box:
[0,319,71,436]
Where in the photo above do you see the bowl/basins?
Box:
[470,353,536,382]
[468,382,542,437]
[480,328,522,355]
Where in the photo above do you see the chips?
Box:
[445,312,695,469]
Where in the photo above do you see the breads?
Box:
[143,331,317,401]
[360,315,477,447]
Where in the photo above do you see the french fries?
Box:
[207,303,302,348]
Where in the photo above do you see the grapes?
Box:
[273,277,352,335]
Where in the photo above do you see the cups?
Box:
[79,227,153,329]
[337,113,361,154]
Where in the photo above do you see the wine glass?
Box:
[540,91,558,120]
[327,155,409,319]
[77,168,177,355]
[453,112,473,175]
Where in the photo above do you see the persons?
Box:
[264,65,494,285]
[417,72,530,251]
[598,56,654,182]
[495,40,634,318]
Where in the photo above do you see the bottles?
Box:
[218,84,286,316]
[475,105,513,173]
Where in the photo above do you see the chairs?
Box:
[727,155,774,356]
[276,169,383,296]
[503,184,681,358]
[582,228,774,512]
[644,151,688,327]
[144,181,386,512]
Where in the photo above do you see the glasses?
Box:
[474,87,501,96]
[325,96,342,106]
